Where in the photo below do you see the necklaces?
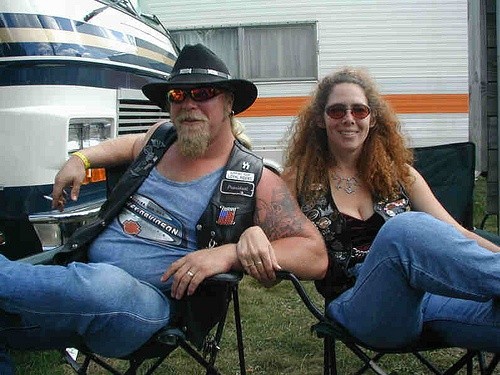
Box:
[329,169,365,194]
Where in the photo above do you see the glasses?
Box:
[324,104,372,119]
[164,86,230,105]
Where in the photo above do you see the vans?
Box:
[1,0,185,262]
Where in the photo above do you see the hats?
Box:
[140,43,259,117]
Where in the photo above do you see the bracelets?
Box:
[72,152,91,169]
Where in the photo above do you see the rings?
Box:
[254,261,262,265]
[247,263,255,266]
[187,271,194,277]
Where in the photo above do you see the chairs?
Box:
[14,162,246,375]
[273,141,500,375]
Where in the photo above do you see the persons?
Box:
[0,43,330,375]
[234,67,500,354]
